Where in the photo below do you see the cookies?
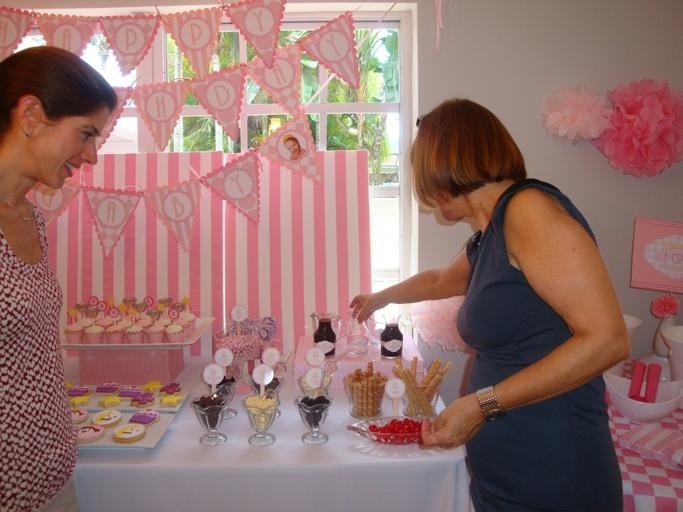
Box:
[344,362,386,417]
[393,356,452,417]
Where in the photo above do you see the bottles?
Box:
[311,308,403,357]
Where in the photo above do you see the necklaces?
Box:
[0,197,34,224]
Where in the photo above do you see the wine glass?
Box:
[193,359,334,447]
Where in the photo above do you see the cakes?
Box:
[65,296,195,343]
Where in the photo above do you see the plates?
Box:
[346,416,422,449]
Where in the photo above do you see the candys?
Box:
[64,380,183,444]
[369,418,422,446]
[194,333,331,431]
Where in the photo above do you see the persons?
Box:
[349,98,631,512]
[0,47,117,512]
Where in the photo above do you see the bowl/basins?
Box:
[602,374,681,423]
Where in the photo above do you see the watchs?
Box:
[476,385,503,422]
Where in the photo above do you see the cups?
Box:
[344,372,443,419]
